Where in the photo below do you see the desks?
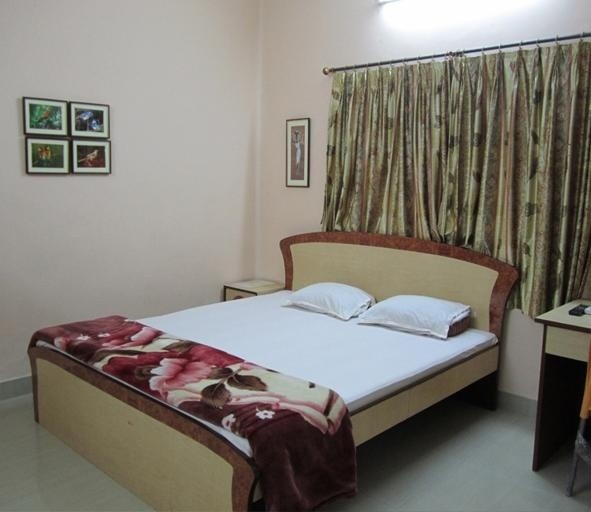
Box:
[532,299,591,472]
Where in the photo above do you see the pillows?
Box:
[448,317,471,336]
[357,295,472,340]
[280,282,377,321]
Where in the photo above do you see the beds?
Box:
[28,232,519,512]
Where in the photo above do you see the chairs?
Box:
[565,336,591,497]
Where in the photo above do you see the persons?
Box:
[294,130,302,169]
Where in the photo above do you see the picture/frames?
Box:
[286,118,310,188]
[71,139,111,174]
[25,136,71,175]
[22,96,70,137]
[69,101,110,139]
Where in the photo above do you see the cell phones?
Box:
[568,303,588,316]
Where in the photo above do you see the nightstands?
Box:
[223,277,284,301]
